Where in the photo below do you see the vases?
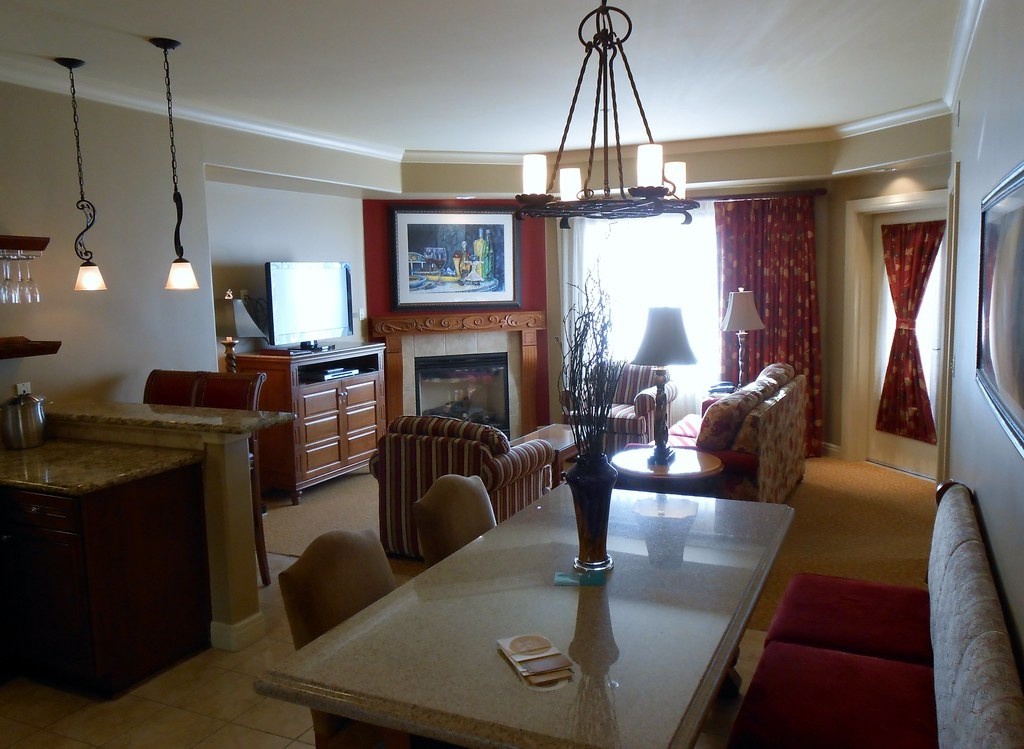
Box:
[566,452,618,572]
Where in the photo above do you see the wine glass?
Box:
[20,255,41,303]
[0,254,13,304]
[10,255,31,304]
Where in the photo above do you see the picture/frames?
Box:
[975,161,1024,457]
[387,203,522,310]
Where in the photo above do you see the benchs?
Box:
[725,479,1024,749]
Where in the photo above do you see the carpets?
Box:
[262,457,938,631]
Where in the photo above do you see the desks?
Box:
[255,484,795,749]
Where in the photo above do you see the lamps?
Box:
[515,0,700,229]
[630,308,698,465]
[54,58,107,290]
[720,287,766,389]
[150,37,199,291]
[215,289,266,372]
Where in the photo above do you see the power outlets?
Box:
[240,290,249,305]
[16,382,31,395]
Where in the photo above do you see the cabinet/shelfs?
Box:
[1,462,211,702]
[235,342,386,507]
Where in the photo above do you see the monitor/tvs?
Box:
[264,261,354,352]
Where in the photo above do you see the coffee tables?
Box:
[509,423,607,488]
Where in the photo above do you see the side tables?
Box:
[610,447,725,495]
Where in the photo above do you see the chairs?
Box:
[279,529,410,748]
[412,474,497,568]
[143,369,271,586]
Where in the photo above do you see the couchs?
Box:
[558,361,678,460]
[623,363,806,504]
[369,416,556,559]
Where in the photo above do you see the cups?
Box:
[0,394,45,450]
